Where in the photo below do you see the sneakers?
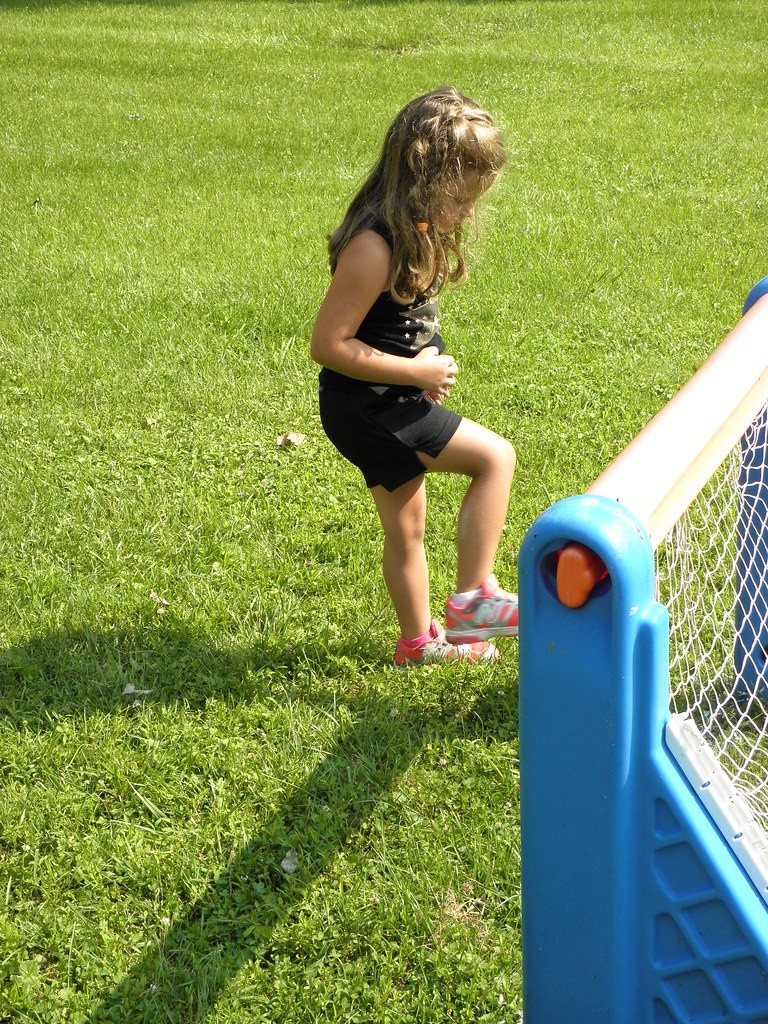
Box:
[395,620,500,667]
[445,575,519,646]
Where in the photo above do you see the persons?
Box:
[310,85,518,667]
